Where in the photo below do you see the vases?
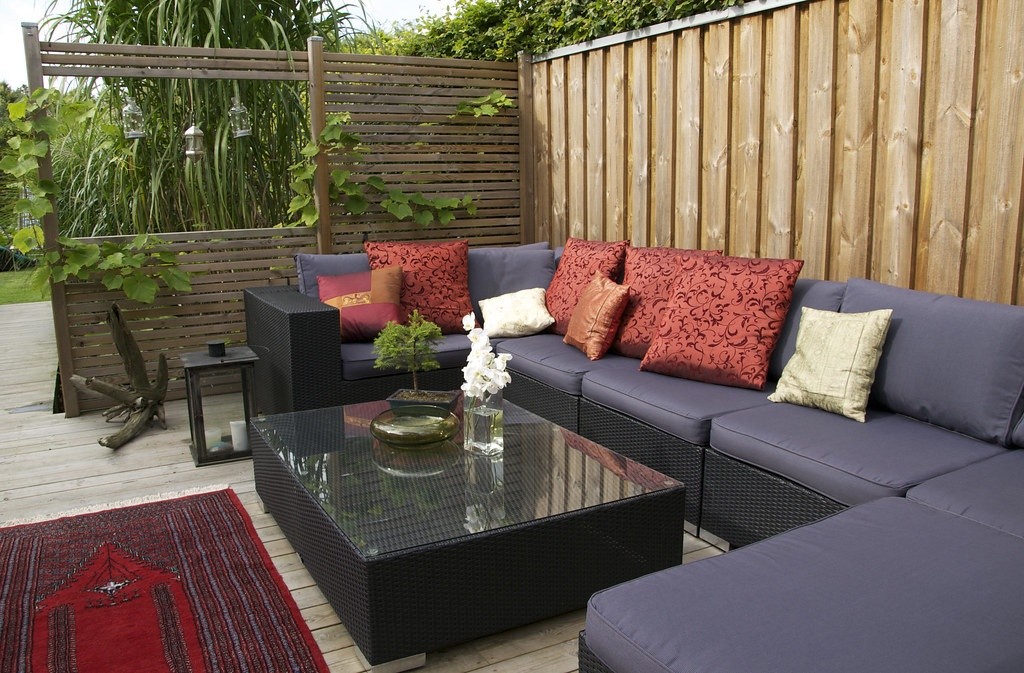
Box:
[462,390,503,457]
[464,453,505,520]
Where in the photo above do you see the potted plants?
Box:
[370,309,463,421]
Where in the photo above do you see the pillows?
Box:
[477,286,556,339]
[611,244,724,358]
[637,251,807,391]
[562,268,631,362]
[543,237,630,335]
[364,238,482,335]
[315,265,403,342]
[766,303,895,423]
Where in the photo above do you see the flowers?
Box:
[463,501,507,534]
[458,310,513,401]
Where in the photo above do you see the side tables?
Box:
[178,345,261,467]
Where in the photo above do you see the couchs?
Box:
[295,236,1024,673]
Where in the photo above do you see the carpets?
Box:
[0,487,331,673]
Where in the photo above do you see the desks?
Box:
[252,389,686,673]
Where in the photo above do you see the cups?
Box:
[230,421,248,451]
[462,387,504,456]
[465,453,505,522]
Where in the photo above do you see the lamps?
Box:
[182,112,205,170]
